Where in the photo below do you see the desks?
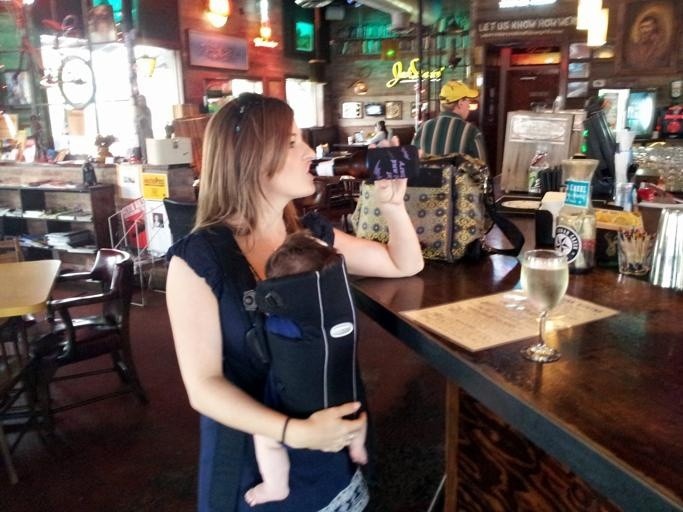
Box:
[344,247,682,510]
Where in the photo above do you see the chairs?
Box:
[1,235,147,487]
[300,178,355,233]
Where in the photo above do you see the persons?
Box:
[630,15,664,71]
[166,91,423,512]
[367,121,390,145]
[243,232,368,509]
[411,81,484,160]
[31,115,43,134]
[135,95,153,164]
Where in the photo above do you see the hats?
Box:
[438,79,479,104]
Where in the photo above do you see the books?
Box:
[22,208,93,222]
[44,230,88,247]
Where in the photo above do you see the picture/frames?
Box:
[564,41,592,98]
[186,29,249,73]
[340,100,401,121]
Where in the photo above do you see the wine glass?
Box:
[519,248,570,362]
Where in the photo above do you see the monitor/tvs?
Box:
[582,111,618,197]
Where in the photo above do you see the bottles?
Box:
[549,159,599,273]
[82,159,98,187]
[309,147,419,183]
[528,150,550,197]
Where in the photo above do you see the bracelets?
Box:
[281,417,290,442]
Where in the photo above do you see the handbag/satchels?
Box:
[350,151,527,265]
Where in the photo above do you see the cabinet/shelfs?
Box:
[325,29,468,61]
[0,178,118,286]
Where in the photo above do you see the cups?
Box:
[618,207,683,288]
